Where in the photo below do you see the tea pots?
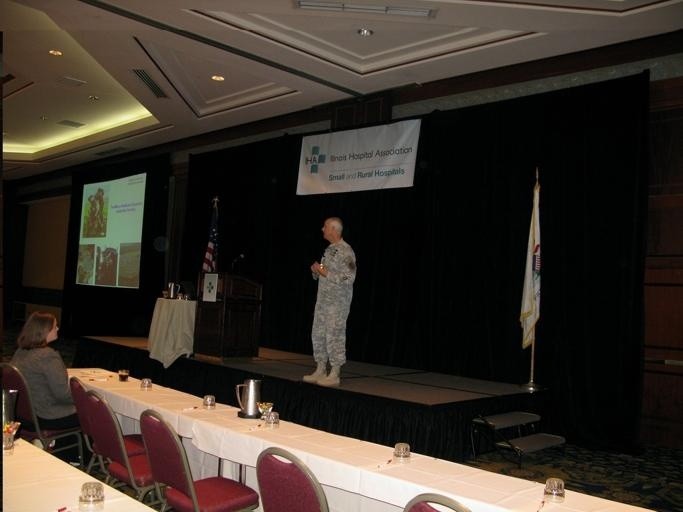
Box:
[236,378,263,419]
[167,281,181,299]
[2,388,19,428]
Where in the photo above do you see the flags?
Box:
[199,205,219,297]
[520,181,541,351]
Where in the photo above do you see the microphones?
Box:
[233,254,245,263]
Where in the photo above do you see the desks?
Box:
[67,367,658,512]
[147,297,197,370]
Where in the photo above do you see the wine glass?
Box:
[259,402,273,425]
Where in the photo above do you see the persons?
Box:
[9,310,95,466]
[302,217,357,386]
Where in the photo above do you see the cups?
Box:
[266,412,279,427]
[140,379,152,390]
[203,395,216,409]
[3,432,15,452]
[543,477,566,503]
[79,481,104,511]
[162,291,191,300]
[393,443,410,464]
[118,370,129,382]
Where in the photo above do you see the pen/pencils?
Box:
[182,407,198,411]
[90,379,108,381]
[378,460,391,468]
[537,501,545,512]
[249,424,261,430]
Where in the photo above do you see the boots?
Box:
[301,363,328,384]
[315,364,342,387]
